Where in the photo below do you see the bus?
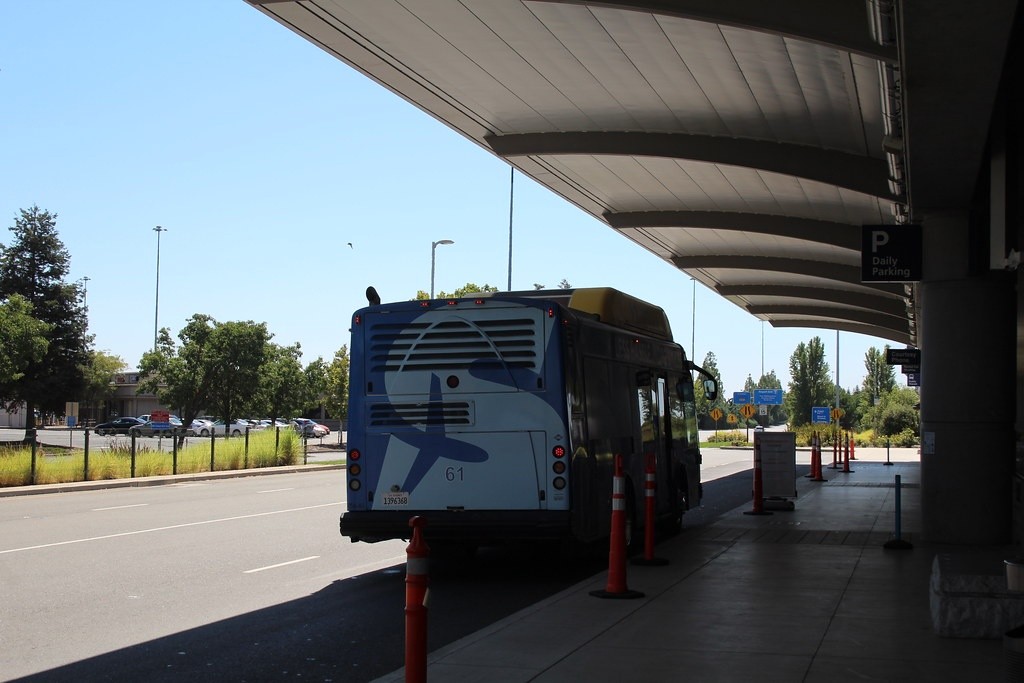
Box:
[339,285,719,544]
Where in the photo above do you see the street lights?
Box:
[82,276,91,353]
[430,240,455,299]
[152,224,168,353]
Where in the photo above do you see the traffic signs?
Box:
[753,389,783,405]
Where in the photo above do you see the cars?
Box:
[94,413,331,438]
